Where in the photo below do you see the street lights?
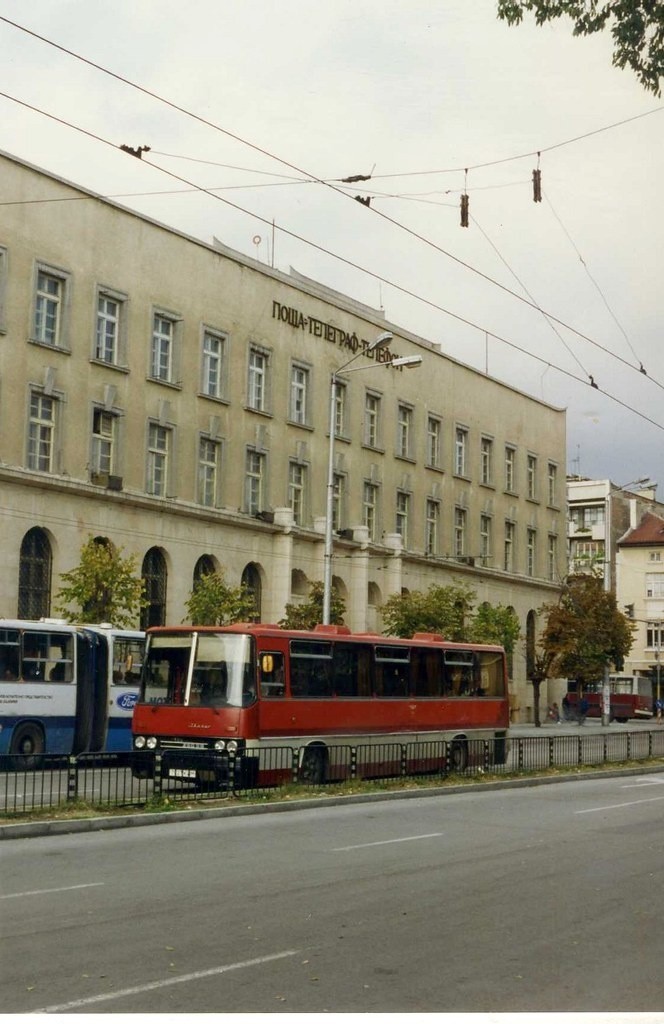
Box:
[323,332,425,620]
[604,476,657,593]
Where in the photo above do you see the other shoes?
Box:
[557,721,562,725]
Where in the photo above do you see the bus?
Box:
[563,674,655,724]
[134,622,511,787]
[1,617,150,767]
